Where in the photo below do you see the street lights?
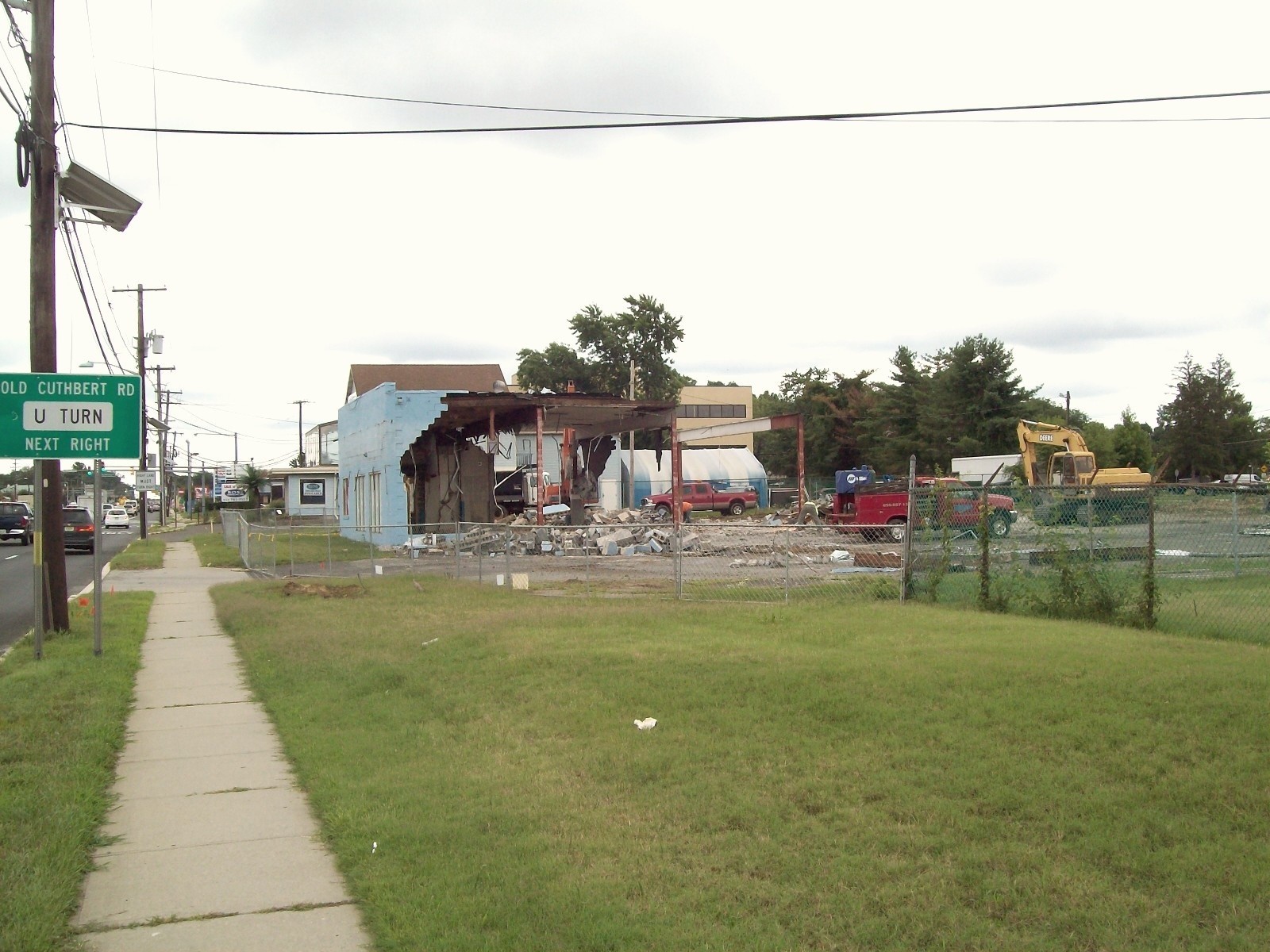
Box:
[194,431,239,464]
[75,359,151,540]
[156,439,192,518]
[1059,390,1070,428]
[189,453,199,480]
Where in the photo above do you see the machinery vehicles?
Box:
[1015,417,1157,527]
[493,377,576,520]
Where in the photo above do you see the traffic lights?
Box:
[130,466,135,475]
[101,462,105,472]
[88,466,92,476]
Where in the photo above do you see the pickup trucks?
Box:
[640,481,757,519]
[831,477,1020,543]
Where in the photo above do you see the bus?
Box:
[125,500,138,511]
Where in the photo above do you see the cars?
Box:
[105,508,130,529]
[148,500,161,513]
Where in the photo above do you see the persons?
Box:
[682,502,693,523]
[797,502,824,533]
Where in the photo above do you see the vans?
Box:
[1224,473,1265,484]
[103,504,114,517]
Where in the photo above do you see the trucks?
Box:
[76,496,104,522]
[84,488,108,505]
[951,453,1065,489]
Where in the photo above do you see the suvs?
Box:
[123,504,137,517]
[0,502,34,546]
[61,505,94,554]
